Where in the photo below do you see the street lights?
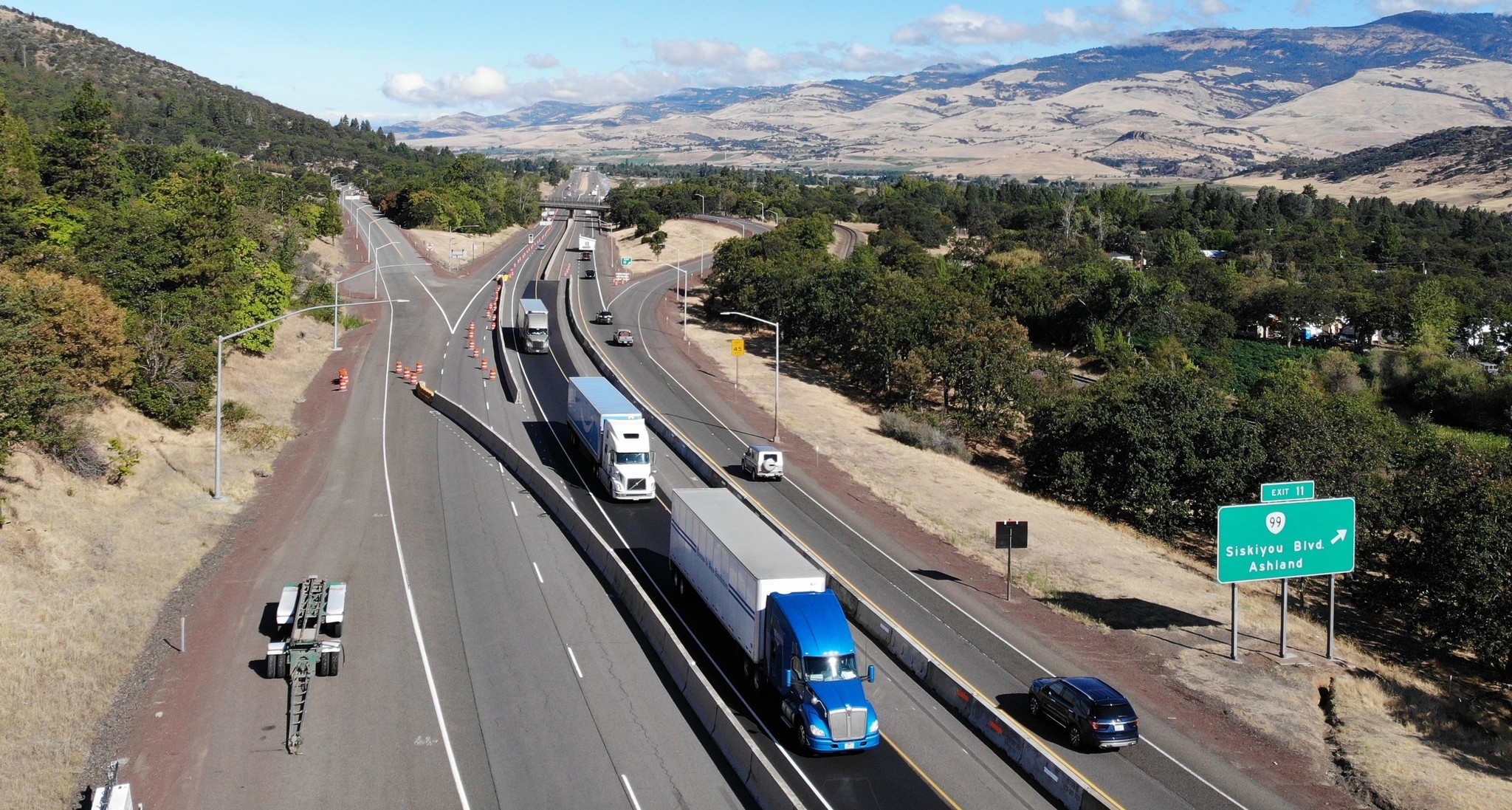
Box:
[329,172,433,351]
[766,210,778,226]
[214,299,411,501]
[720,312,780,444]
[586,217,614,267]
[448,225,479,271]
[690,235,703,278]
[658,264,687,340]
[753,201,764,225]
[730,221,745,239]
[695,194,704,215]
[658,244,680,300]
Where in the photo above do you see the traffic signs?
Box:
[1217,496,1356,584]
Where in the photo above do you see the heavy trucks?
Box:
[518,299,552,355]
[567,377,657,502]
[668,487,880,754]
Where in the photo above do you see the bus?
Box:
[1029,675,1140,750]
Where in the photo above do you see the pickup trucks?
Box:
[613,329,634,347]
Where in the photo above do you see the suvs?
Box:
[596,310,613,325]
[741,446,784,482]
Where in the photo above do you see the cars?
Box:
[563,183,598,197]
[537,243,546,251]
[581,252,592,261]
[585,269,595,280]
